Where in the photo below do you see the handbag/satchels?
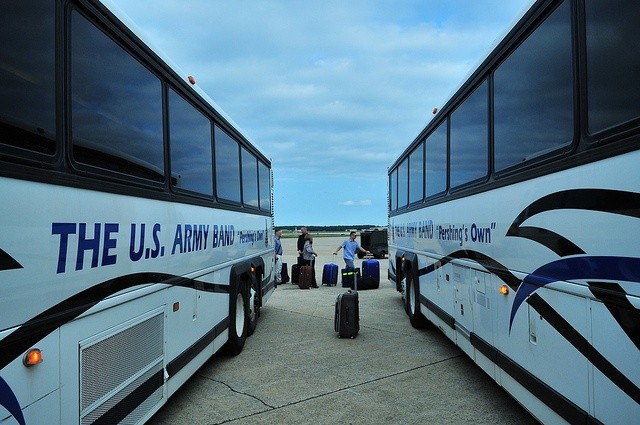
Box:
[351,276,378,290]
[342,267,360,288]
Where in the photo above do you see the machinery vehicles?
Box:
[357,228,388,259]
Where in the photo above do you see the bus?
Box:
[0,0,277,425]
[386,0,640,425]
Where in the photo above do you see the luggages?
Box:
[322,252,338,286]
[292,253,303,285]
[275,257,278,288]
[362,252,379,289]
[281,263,289,282]
[334,270,359,339]
[298,253,313,289]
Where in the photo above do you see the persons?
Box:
[297,226,308,264]
[274,228,283,284]
[334,231,369,271]
[303,234,319,288]
